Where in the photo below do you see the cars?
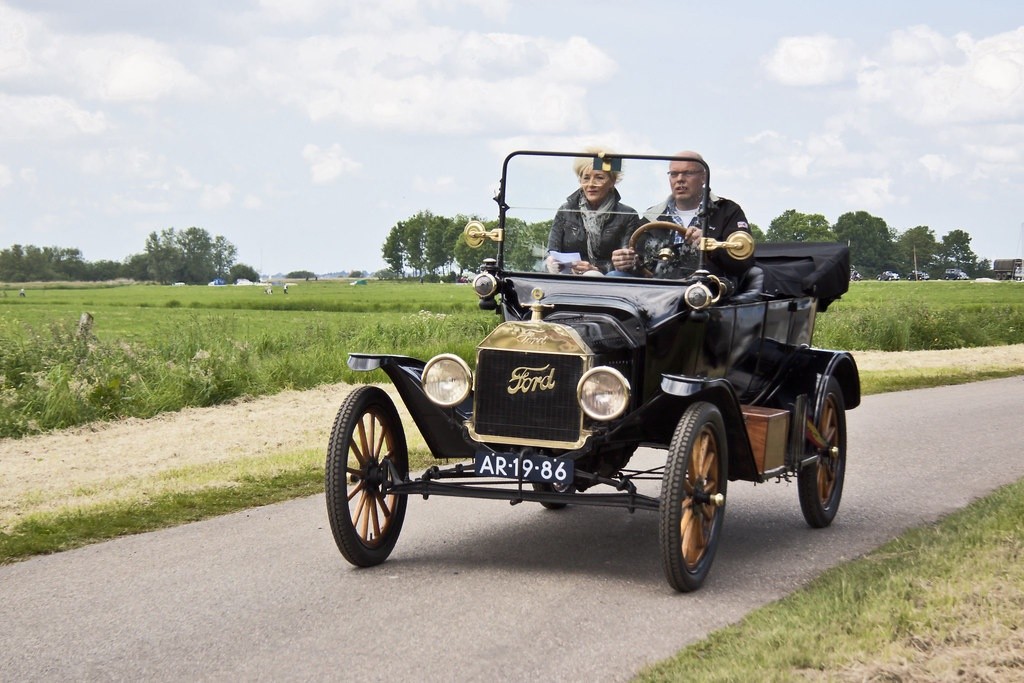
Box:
[458,276,469,283]
[323,149,862,592]
[907,270,929,280]
[876,270,900,281]
[849,264,861,281]
[944,268,970,280]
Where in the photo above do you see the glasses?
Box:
[667,170,704,177]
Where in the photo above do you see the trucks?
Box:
[993,258,1024,282]
[350,280,367,286]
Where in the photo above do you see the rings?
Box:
[579,270,583,275]
[691,225,696,232]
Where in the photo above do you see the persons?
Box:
[283,282,289,294]
[531,148,641,279]
[604,150,756,298]
